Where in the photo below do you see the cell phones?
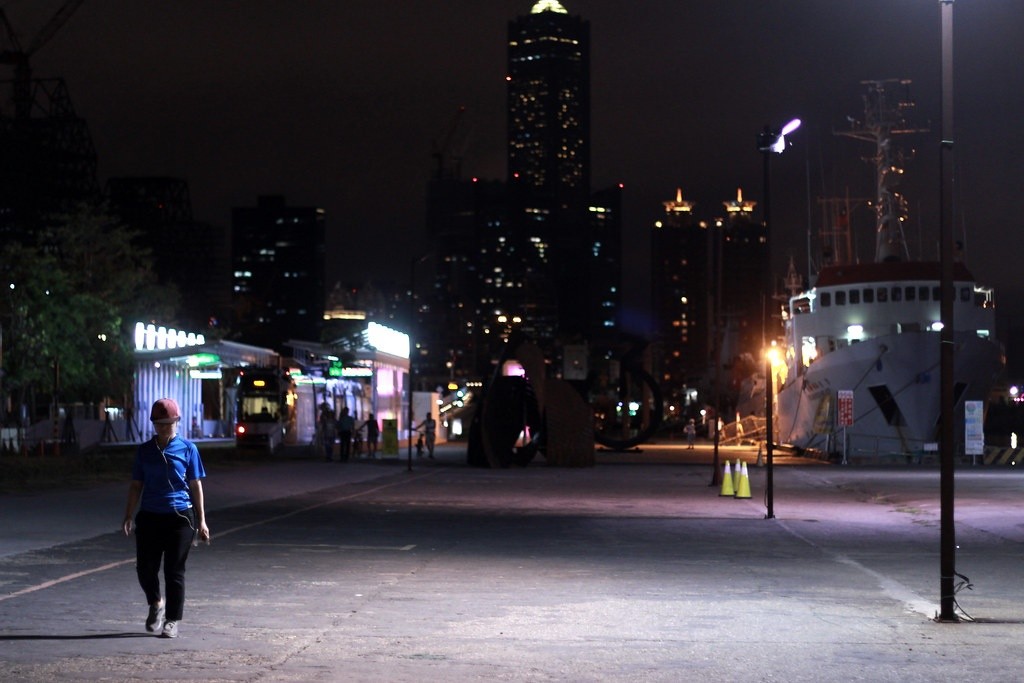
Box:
[202,531,209,544]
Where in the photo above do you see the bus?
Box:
[233,370,282,455]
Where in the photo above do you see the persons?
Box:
[123,399,210,638]
[416,412,436,458]
[686,418,695,450]
[318,402,379,463]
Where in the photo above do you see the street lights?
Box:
[758,116,802,518]
[937,1,963,626]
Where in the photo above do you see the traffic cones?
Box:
[734,461,752,499]
[1,436,61,458]
[733,458,741,492]
[719,460,736,496]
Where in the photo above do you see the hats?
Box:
[149,397,180,424]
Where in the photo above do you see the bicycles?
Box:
[351,427,364,456]
[413,428,427,460]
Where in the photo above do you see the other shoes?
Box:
[146,603,165,631]
[162,620,178,638]
